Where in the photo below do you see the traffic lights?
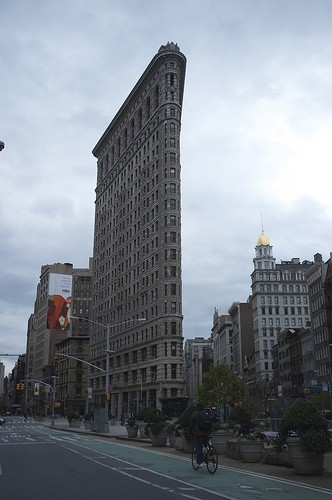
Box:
[54,357,59,367]
[16,384,20,390]
[21,384,24,390]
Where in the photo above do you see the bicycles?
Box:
[188,433,219,475]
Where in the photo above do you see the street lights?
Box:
[70,315,147,433]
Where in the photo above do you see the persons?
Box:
[24,411,29,423]
[187,403,212,467]
